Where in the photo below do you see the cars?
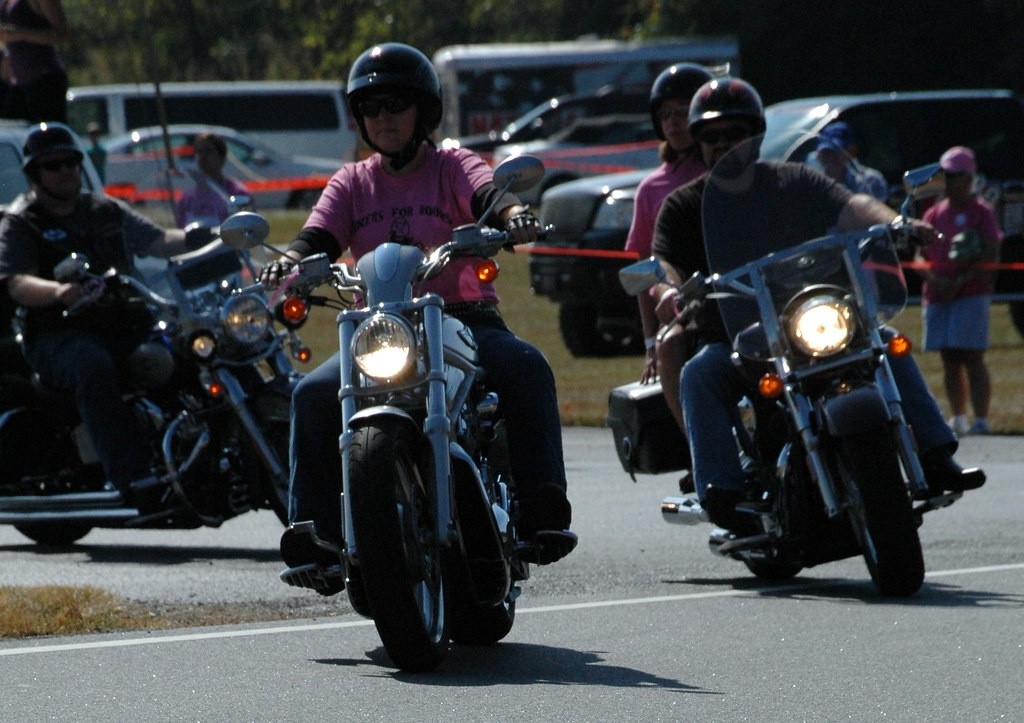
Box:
[531,92,1024,359]
[0,126,105,205]
[89,123,347,211]
[437,85,676,222]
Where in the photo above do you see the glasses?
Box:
[655,102,689,120]
[697,124,759,144]
[30,156,81,179]
[356,90,421,118]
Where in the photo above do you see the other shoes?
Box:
[516,511,577,567]
[129,467,174,530]
[944,414,968,435]
[966,418,990,434]
[679,473,697,494]
[279,525,346,597]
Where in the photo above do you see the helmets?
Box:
[648,61,713,141]
[20,121,84,172]
[688,76,767,139]
[347,41,443,134]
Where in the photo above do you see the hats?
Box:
[818,122,854,152]
[938,144,977,178]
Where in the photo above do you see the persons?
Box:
[257,41,581,597]
[84,123,106,186]
[174,128,255,314]
[622,62,1004,552]
[0,122,222,492]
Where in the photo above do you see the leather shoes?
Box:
[911,450,987,500]
[705,483,763,539]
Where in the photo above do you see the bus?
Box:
[435,39,743,140]
[61,82,374,211]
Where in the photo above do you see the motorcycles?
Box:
[219,155,559,675]
[0,193,306,544]
[607,164,989,598]
